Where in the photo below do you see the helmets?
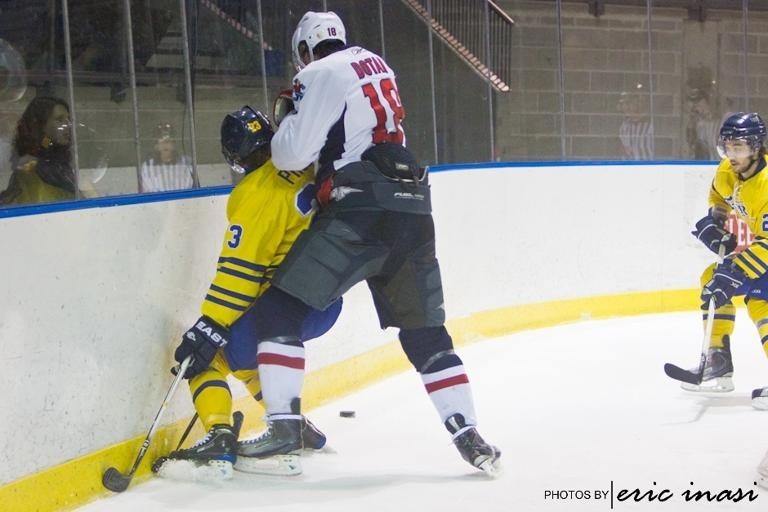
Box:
[153,123,178,145]
[291,11,346,72]
[48,122,109,188]
[716,111,766,159]
[220,105,274,177]
[0,38,28,104]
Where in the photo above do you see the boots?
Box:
[752,386,768,399]
[445,413,501,471]
[237,397,326,457]
[151,411,243,472]
[689,335,733,381]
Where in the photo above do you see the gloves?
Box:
[170,314,231,379]
[700,263,746,310]
[273,89,296,127]
[691,216,737,255]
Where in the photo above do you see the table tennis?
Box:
[102,355,215,492]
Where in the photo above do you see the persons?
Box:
[3,95,87,203]
[680,76,737,159]
[170,105,311,458]
[141,120,191,192]
[237,10,499,457]
[618,82,667,161]
[687,110,768,397]
